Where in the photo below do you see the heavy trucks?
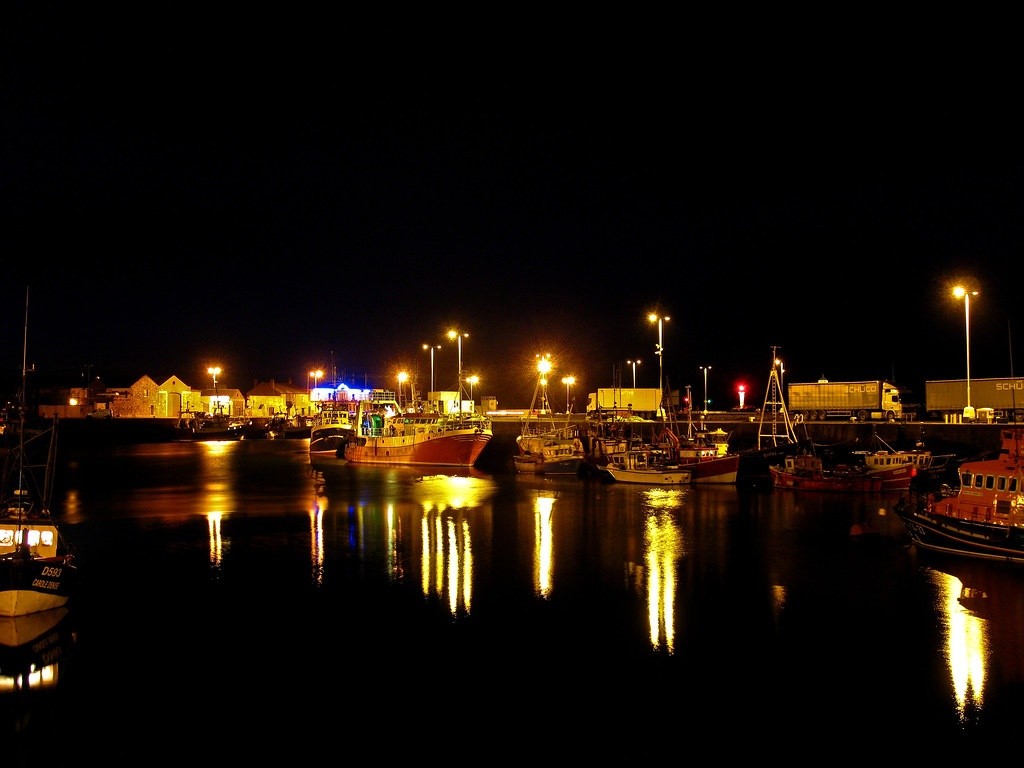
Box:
[788,380,902,422]
[586,387,660,420]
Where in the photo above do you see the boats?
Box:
[174,393,354,454]
[891,318,1024,563]
[0,281,80,617]
[342,398,495,467]
[512,357,966,494]
[0,604,78,695]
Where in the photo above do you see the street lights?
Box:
[627,359,641,388]
[562,376,575,414]
[954,289,980,423]
[467,377,477,400]
[449,331,470,425]
[422,345,442,392]
[699,366,711,410]
[310,372,321,388]
[649,315,671,401]
[209,369,220,389]
